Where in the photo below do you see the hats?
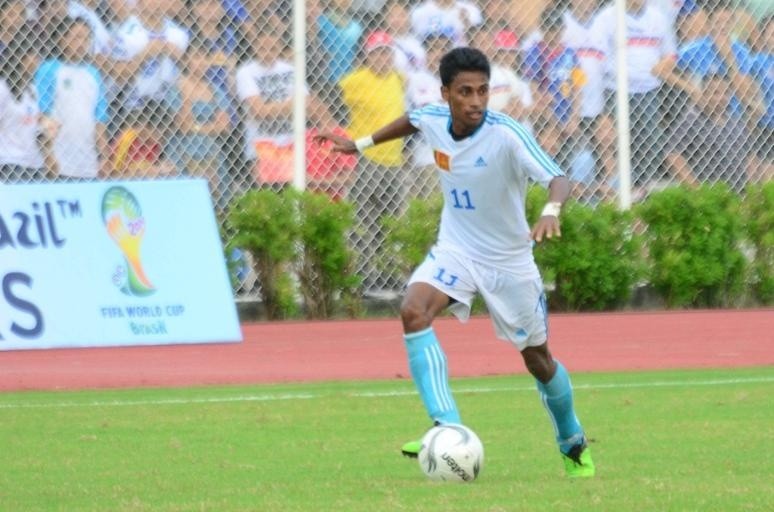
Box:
[492,28,522,52]
[363,30,399,55]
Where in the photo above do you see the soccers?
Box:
[417,423,483,482]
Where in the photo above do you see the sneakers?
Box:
[400,437,424,460]
[559,439,597,481]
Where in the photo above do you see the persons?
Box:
[313,47,598,483]
[0,2,774,293]
